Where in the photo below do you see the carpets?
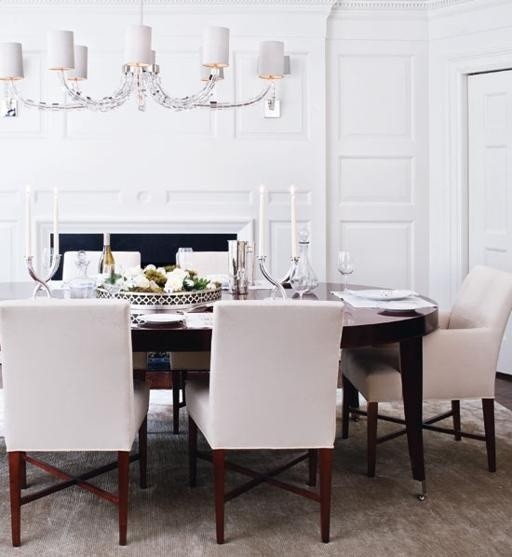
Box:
[0,390,512,557]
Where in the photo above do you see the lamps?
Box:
[0,0,285,117]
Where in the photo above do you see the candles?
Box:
[53,199,59,254]
[259,194,268,256]
[26,197,32,255]
[291,196,298,257]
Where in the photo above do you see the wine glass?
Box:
[288,268,313,300]
[338,248,355,291]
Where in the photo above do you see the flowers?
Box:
[105,264,218,292]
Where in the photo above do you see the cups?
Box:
[178,248,192,271]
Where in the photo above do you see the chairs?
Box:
[340,264,511,478]
[62,252,142,279]
[185,298,345,545]
[176,252,256,287]
[1,296,151,546]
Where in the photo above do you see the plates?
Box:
[138,314,187,323]
[349,289,415,300]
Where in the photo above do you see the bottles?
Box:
[290,226,319,294]
[98,232,115,277]
